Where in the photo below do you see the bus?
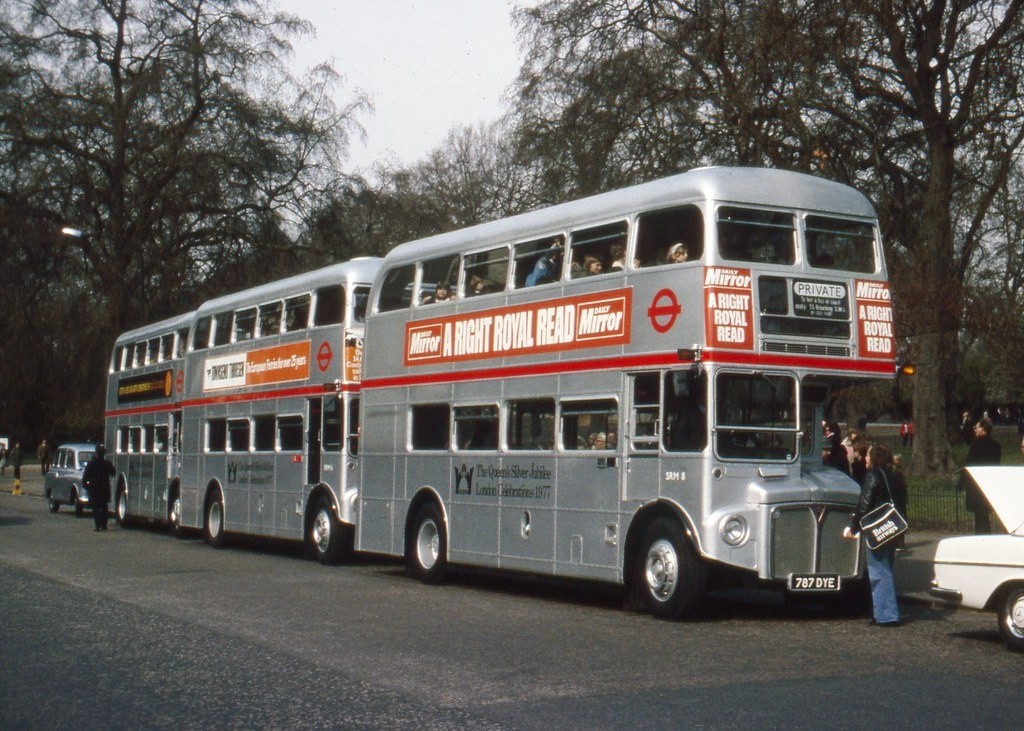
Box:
[105,313,198,540]
[178,255,458,561]
[355,165,915,620]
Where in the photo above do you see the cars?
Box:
[43,442,101,517]
[929,466,1024,652]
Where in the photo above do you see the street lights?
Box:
[64,229,129,330]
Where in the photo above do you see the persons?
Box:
[85,436,92,444]
[0,443,9,476]
[900,413,914,451]
[842,446,909,627]
[820,406,859,478]
[463,401,814,461]
[9,442,23,500]
[419,237,689,307]
[37,440,51,476]
[82,443,116,533]
[955,407,1024,536]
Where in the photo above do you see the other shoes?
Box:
[869,619,900,625]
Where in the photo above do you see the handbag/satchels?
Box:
[859,502,909,551]
